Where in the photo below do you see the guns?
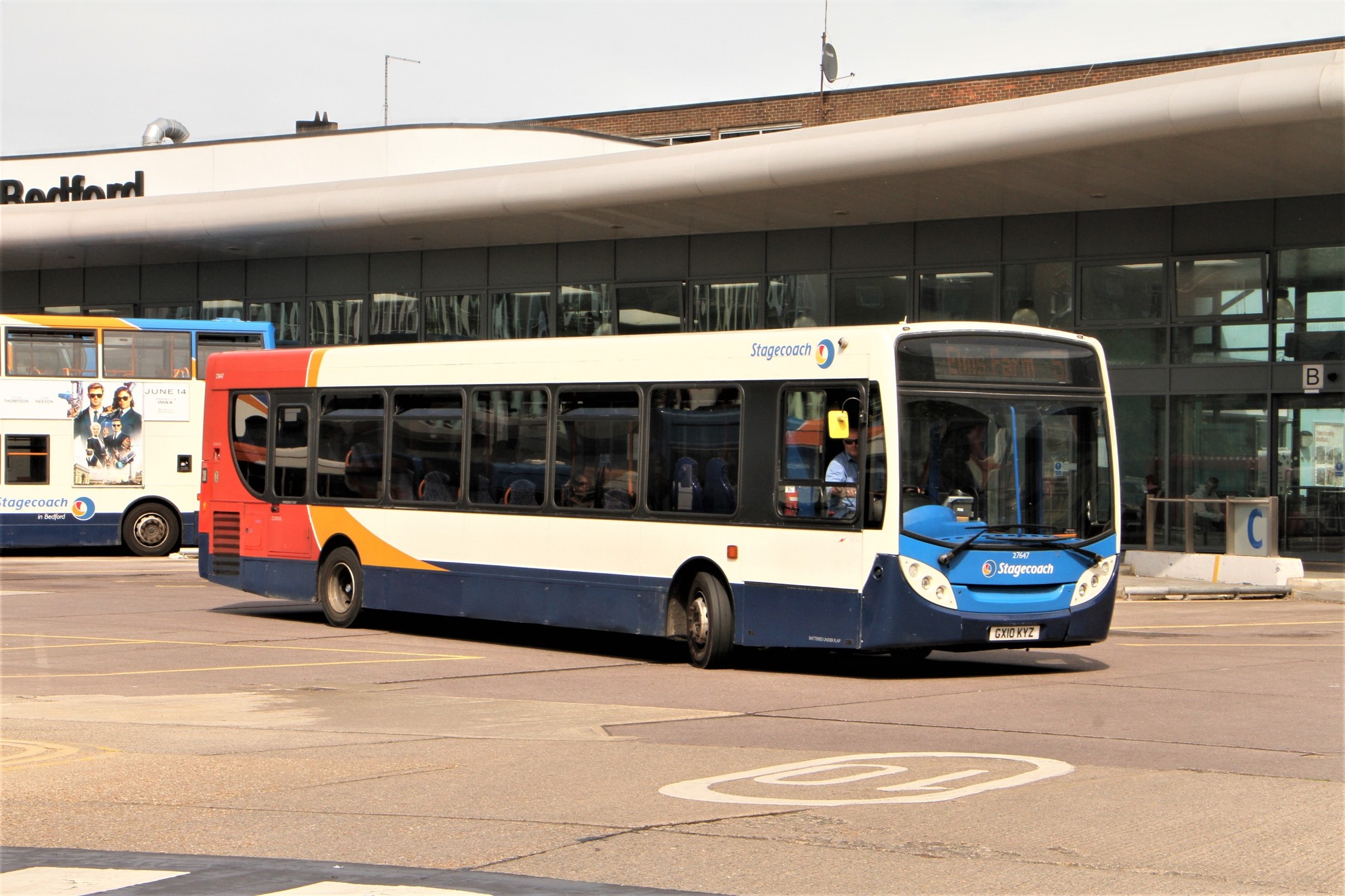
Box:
[103,382,138,418]
[58,381,83,416]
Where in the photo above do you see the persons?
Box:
[1121,474,1166,540]
[1191,477,1225,546]
[67,382,110,445]
[919,413,1012,505]
[98,386,143,446]
[102,419,131,456]
[825,423,867,513]
[86,422,106,468]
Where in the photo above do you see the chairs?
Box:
[242,415,737,515]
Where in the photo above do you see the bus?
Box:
[0,312,276,557]
[198,319,1121,670]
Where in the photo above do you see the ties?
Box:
[94,411,98,422]
[114,433,117,440]
[119,410,123,417]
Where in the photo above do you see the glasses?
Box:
[89,394,103,398]
[118,396,128,401]
[843,439,858,445]
[112,424,121,427]
